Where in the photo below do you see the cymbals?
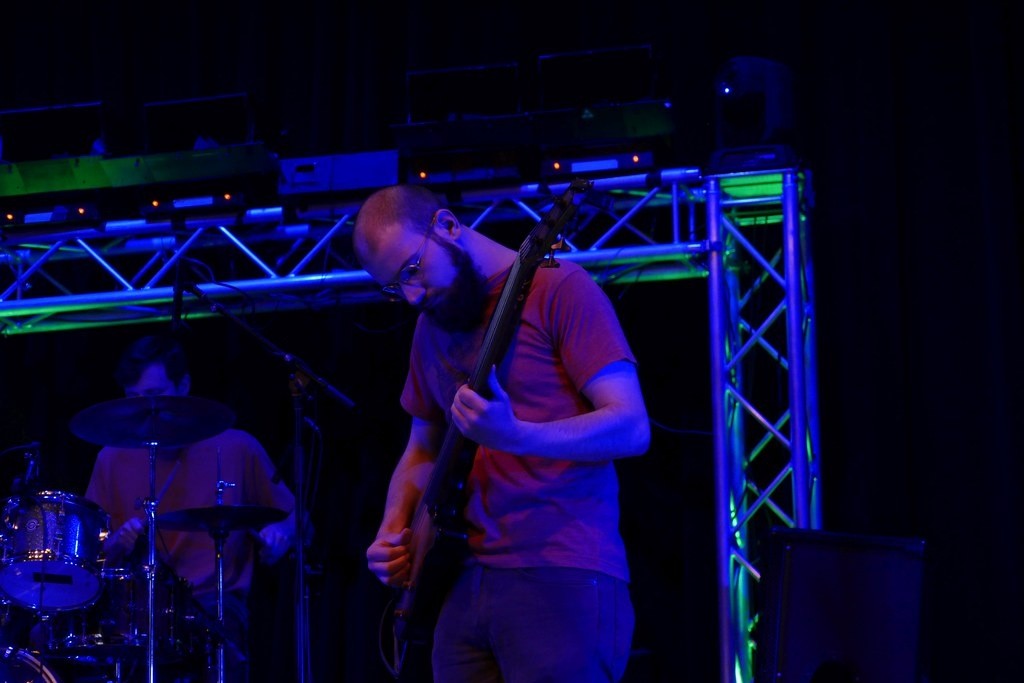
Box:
[154,504,289,533]
[68,394,236,449]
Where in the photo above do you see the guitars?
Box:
[375,176,597,683]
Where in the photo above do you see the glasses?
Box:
[380,223,435,299]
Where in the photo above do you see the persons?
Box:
[354,186,652,683]
[78,337,312,683]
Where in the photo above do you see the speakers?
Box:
[270,130,401,195]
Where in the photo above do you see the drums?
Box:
[52,639,187,681]
[112,557,181,632]
[0,642,58,683]
[1,490,114,613]
